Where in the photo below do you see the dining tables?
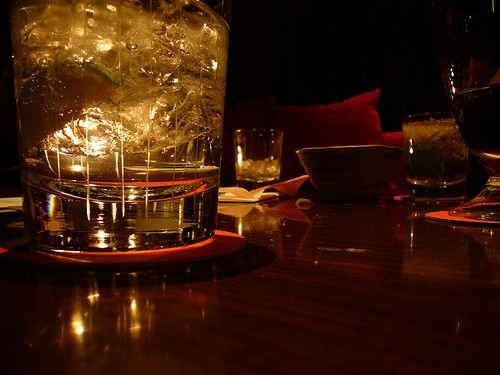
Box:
[1,187,499,374]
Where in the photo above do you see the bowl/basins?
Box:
[296,145,399,203]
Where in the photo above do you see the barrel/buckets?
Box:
[11,0,231,253]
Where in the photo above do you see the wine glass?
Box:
[432,0,500,223]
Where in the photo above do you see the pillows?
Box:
[267,88,384,183]
[222,95,278,182]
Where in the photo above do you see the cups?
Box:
[401,109,469,206]
[233,128,283,192]
